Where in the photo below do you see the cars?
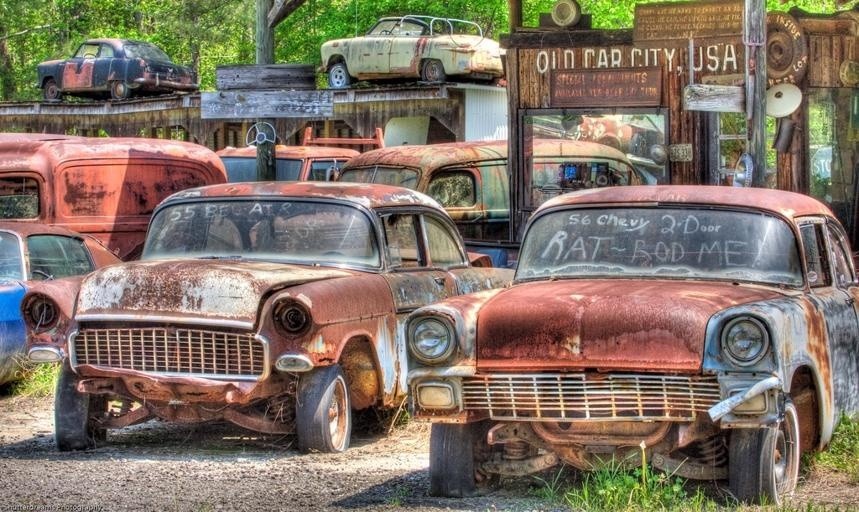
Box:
[403,183,859,503]
[320,15,505,88]
[38,38,201,98]
[0,132,859,269]
[54,180,494,452]
[1,224,125,388]
